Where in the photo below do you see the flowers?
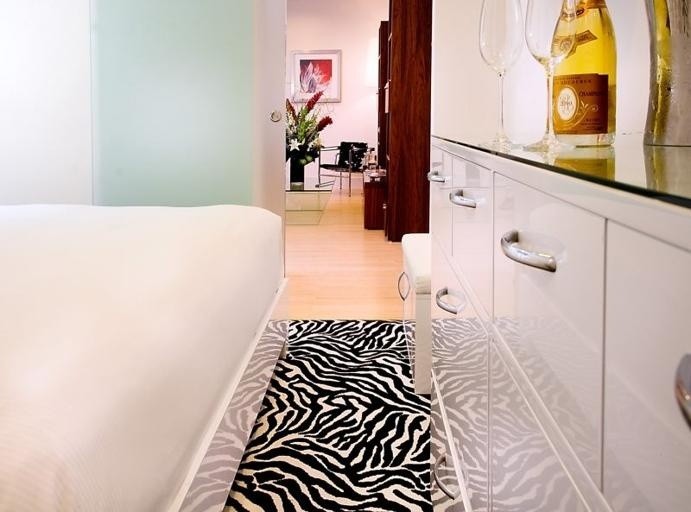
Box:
[286,90,334,166]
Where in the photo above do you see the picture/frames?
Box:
[290,49,341,103]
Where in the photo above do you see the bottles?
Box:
[550,0,617,148]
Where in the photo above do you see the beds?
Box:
[0,202,289,512]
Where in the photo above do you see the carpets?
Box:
[430,315,653,511]
[403,314,416,378]
[180,320,289,512]
[223,317,434,512]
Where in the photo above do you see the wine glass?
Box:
[479,0,525,151]
[523,0,577,154]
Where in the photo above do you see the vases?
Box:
[290,153,305,183]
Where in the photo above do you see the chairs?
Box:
[319,142,369,198]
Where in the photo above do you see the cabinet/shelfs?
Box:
[429,144,691,512]
[364,0,428,241]
[396,233,429,396]
